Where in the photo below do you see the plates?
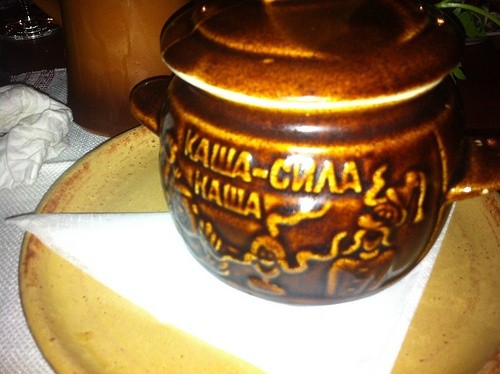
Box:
[18,125,500,373]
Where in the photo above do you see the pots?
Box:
[130,1,500,306]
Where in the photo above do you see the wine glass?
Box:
[2,0,61,40]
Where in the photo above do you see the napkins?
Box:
[3,202,455,374]
